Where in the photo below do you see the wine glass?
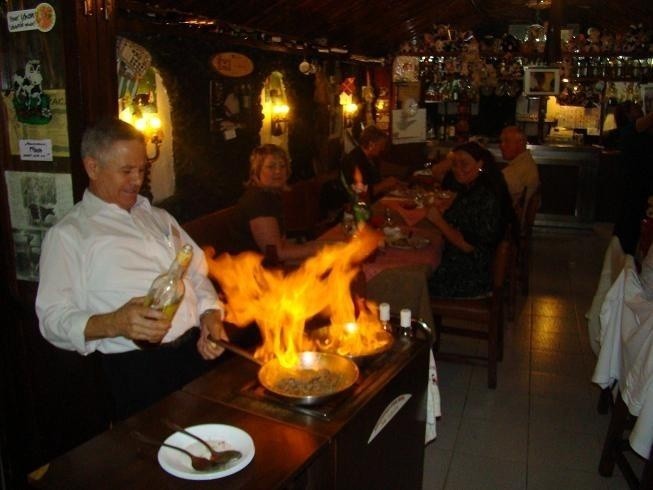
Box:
[341,223,352,239]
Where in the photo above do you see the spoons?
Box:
[129,428,221,472]
[165,420,242,467]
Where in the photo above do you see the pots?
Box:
[204,333,359,407]
[302,322,395,364]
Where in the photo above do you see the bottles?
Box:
[425,117,463,145]
[399,308,415,355]
[378,302,392,334]
[133,243,196,353]
[381,207,393,236]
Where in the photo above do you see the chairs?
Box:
[596,235,653,490]
[432,192,541,391]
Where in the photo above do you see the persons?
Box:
[340,125,399,215]
[426,142,508,300]
[613,99,653,255]
[499,124,540,229]
[34,118,230,425]
[232,143,336,268]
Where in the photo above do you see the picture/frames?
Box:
[523,67,560,96]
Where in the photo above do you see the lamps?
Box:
[402,98,417,129]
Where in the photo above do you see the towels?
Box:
[425,347,442,445]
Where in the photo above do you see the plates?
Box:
[388,235,430,250]
[154,423,257,483]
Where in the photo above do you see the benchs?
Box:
[179,169,336,341]
